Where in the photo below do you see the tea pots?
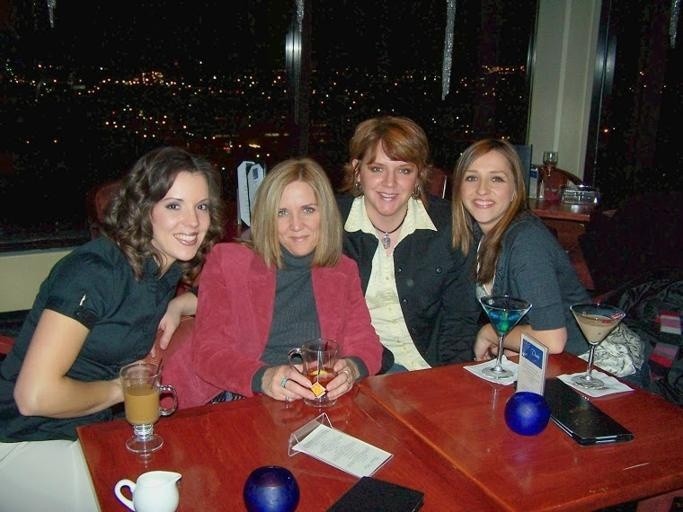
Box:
[114,469,182,512]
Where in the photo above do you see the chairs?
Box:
[518,156,592,213]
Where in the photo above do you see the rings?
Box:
[281,377,287,388]
[340,370,350,379]
[285,396,289,401]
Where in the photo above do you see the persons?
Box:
[157,157,382,411]
[0,146,224,511]
[451,136,653,512]
[151,114,478,375]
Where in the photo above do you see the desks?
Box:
[518,182,614,295]
[74,343,683,511]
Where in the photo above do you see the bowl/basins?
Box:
[559,186,596,204]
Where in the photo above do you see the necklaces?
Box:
[368,206,408,249]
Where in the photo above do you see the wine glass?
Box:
[119,361,177,457]
[543,150,557,177]
[568,301,627,390]
[479,294,531,379]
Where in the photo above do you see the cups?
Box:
[286,339,340,408]
